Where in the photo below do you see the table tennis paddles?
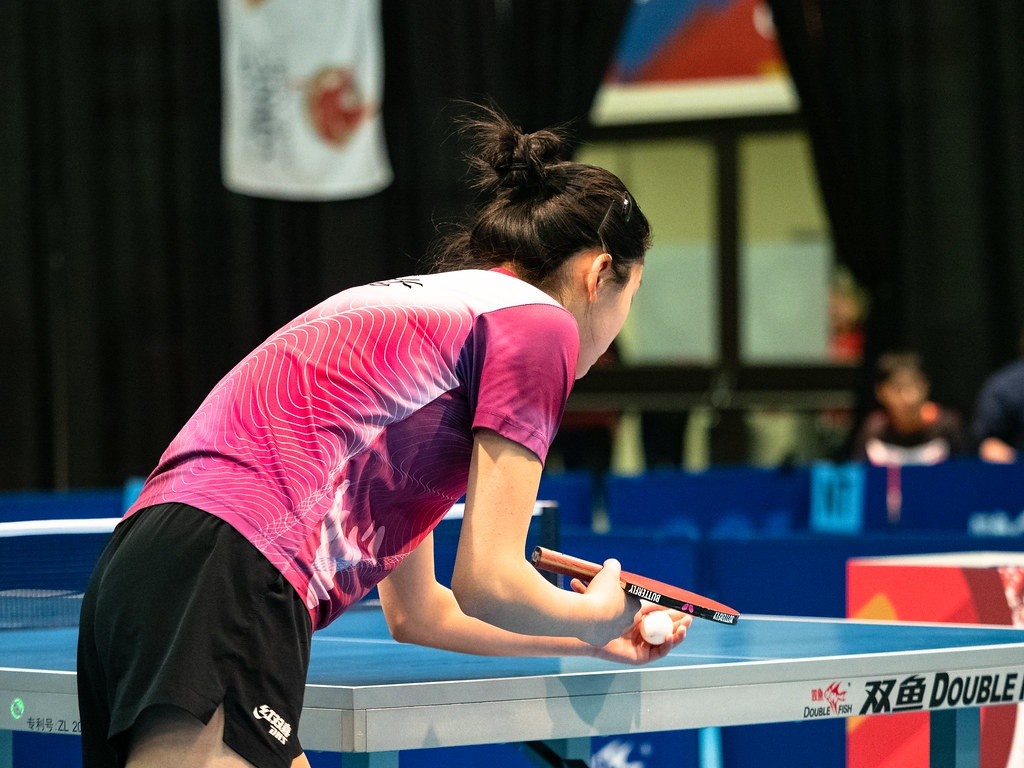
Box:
[529,544,743,627]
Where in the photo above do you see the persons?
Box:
[77,97,693,768]
[847,351,968,522]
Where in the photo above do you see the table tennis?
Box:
[638,609,674,647]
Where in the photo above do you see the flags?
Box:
[219,0,400,204]
